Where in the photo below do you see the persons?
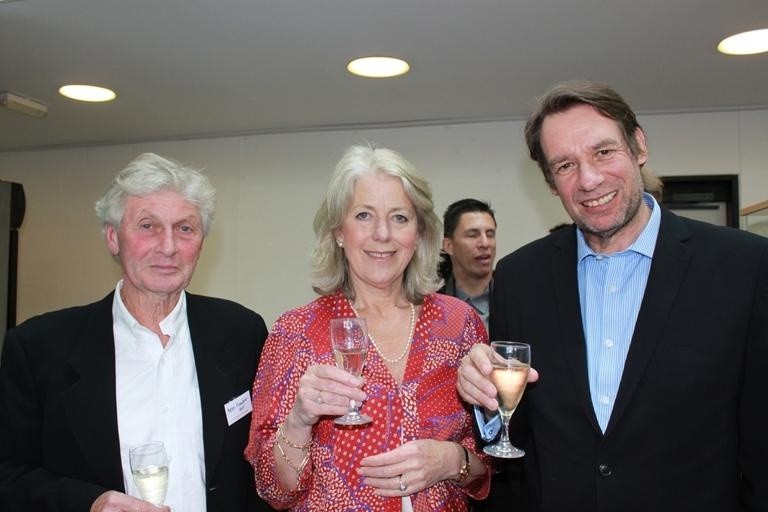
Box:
[457,81,767,510]
[1,152,291,512]
[433,198,498,338]
[244,145,496,512]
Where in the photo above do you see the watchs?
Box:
[454,443,472,484]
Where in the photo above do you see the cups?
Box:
[129,441,169,507]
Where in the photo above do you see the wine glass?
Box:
[481,341,532,458]
[328,318,371,425]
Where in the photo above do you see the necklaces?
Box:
[346,293,415,363]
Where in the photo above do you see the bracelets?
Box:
[275,426,312,492]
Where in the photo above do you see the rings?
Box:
[399,475,407,493]
[316,390,324,405]
